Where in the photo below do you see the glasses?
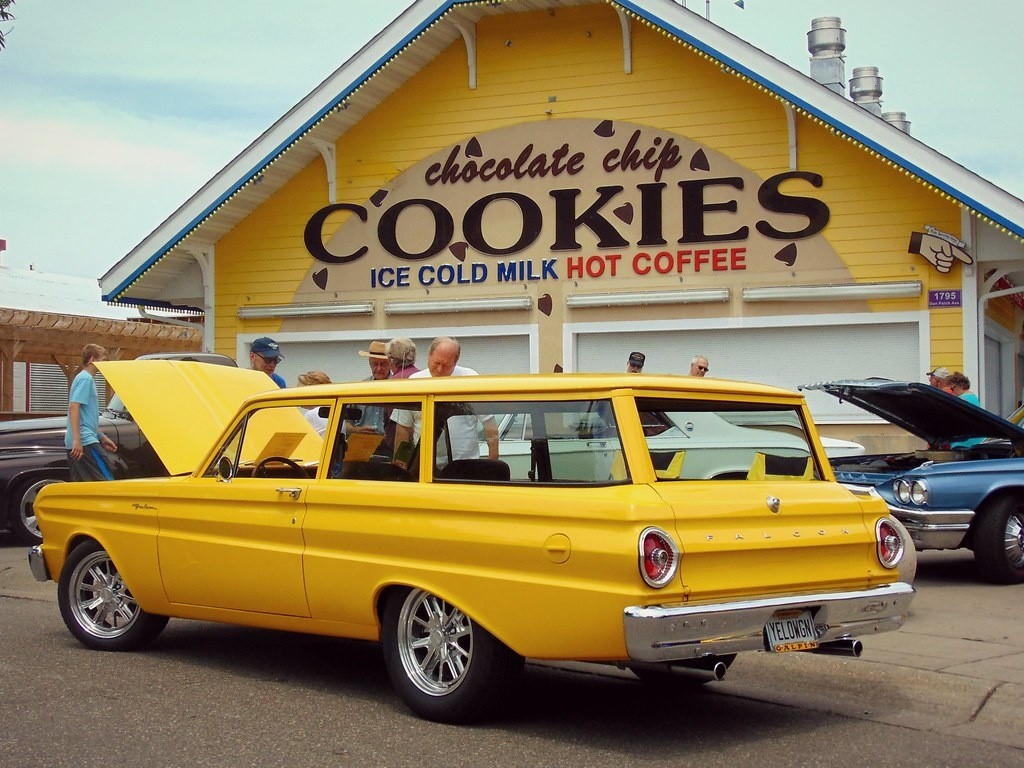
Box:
[255,352,281,364]
[630,363,642,369]
[695,364,709,372]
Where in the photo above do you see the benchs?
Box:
[607,424,668,438]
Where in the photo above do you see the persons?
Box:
[246,337,286,388]
[297,371,346,441]
[65,344,117,482]
[626,351,645,373]
[688,355,709,377]
[924,367,987,450]
[345,337,498,469]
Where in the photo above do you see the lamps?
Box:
[237,300,375,320]
[566,287,731,309]
[384,296,534,316]
[741,280,924,303]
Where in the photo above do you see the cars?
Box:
[791,375,1024,583]
[0,352,241,546]
[476,409,872,486]
[27,359,922,727]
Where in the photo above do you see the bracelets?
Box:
[97,432,104,439]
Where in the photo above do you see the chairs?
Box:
[604,449,687,482]
[335,460,412,482]
[437,458,512,481]
[745,451,816,482]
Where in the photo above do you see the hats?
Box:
[252,337,285,359]
[629,352,645,366]
[359,341,389,359]
[926,367,949,379]
